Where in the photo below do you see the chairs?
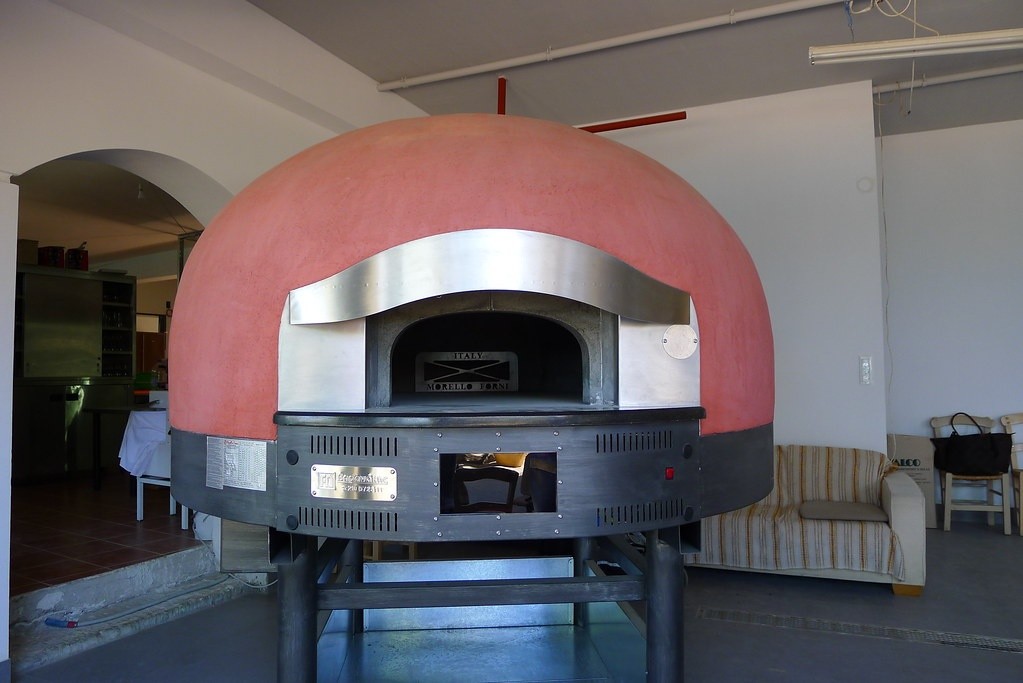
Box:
[1000,412,1023,536]
[930,415,1011,536]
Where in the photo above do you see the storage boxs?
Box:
[886,434,938,529]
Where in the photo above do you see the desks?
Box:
[118,409,189,530]
[83,404,148,491]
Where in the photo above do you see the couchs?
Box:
[683,444,927,597]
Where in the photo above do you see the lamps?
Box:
[808,0,1023,66]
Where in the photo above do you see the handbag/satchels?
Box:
[930,413,1016,476]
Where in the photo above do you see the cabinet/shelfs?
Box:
[102,301,133,354]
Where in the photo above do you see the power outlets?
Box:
[858,355,872,385]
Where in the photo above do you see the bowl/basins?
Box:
[494,453,526,466]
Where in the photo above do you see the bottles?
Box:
[157,366,167,390]
